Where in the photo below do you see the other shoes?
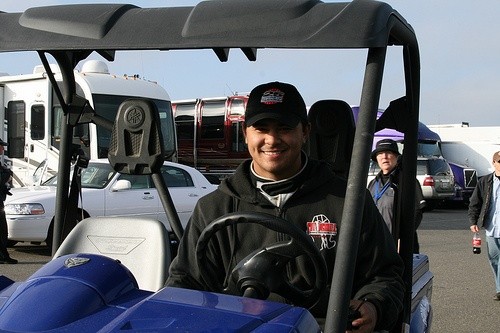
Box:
[0,258,17,264]
[493,292,500,301]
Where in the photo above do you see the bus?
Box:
[353,106,459,209]
[173,93,251,181]
[1,59,179,189]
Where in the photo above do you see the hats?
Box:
[0,138,8,146]
[244,82,308,127]
[371,139,402,162]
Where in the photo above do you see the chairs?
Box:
[51,215,172,292]
[93,172,184,188]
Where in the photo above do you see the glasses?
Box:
[494,160,500,163]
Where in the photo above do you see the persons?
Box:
[468,150,500,301]
[367,139,423,255]
[164,81,399,333]
[0,138,18,264]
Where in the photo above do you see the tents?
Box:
[351,106,441,156]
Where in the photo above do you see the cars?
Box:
[5,158,221,248]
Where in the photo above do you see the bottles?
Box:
[472,228,481,254]
